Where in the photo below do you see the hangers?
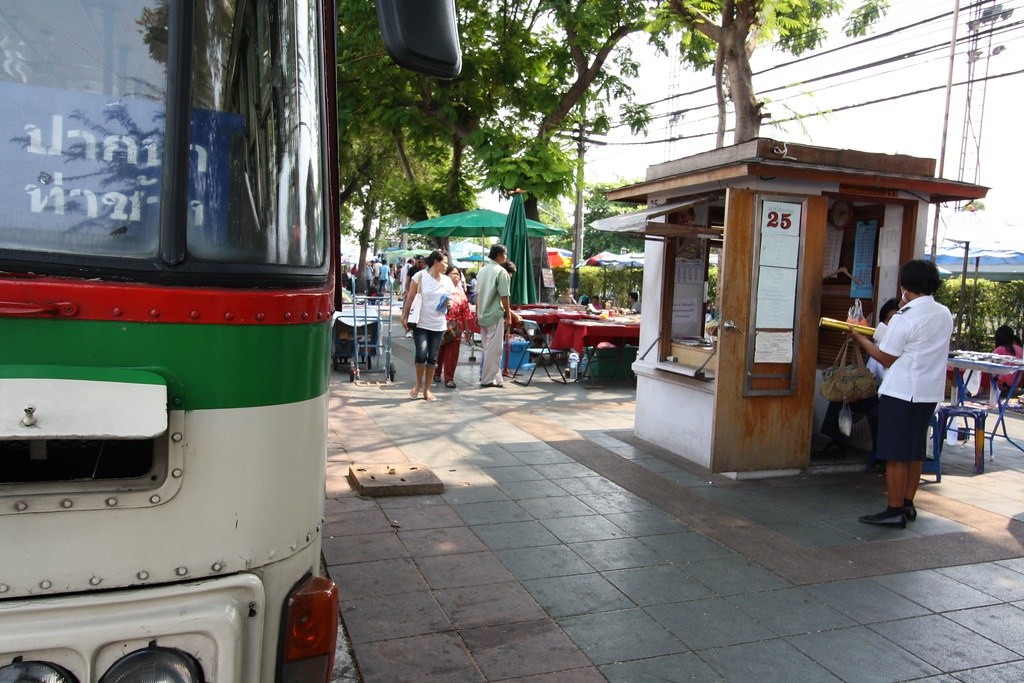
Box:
[823,264,861,285]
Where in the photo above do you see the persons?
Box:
[842,259,953,530]
[628,292,641,313]
[351,259,395,293]
[399,254,428,338]
[476,244,523,388]
[460,268,477,305]
[947,325,1024,394]
[590,296,602,309]
[401,251,468,402]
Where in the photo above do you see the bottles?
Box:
[570,358,577,380]
[580,354,590,377]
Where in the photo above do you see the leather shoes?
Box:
[903,507,916,521]
[865,458,886,474]
[859,511,907,529]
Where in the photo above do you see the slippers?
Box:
[409,384,421,400]
[423,391,436,401]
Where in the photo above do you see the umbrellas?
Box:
[397,209,569,269]
[498,188,538,374]
[546,246,644,313]
[385,242,491,270]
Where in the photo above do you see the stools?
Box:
[921,415,941,483]
[937,403,988,475]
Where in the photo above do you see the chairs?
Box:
[511,318,567,388]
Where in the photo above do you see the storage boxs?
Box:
[500,341,531,369]
[585,347,638,380]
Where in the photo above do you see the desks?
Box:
[331,307,383,370]
[469,309,608,363]
[550,319,640,389]
[932,349,1024,461]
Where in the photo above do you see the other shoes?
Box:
[445,380,456,388]
[433,365,442,382]
[406,330,413,338]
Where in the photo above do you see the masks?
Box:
[901,290,908,303]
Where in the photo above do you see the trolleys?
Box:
[349,277,396,387]
[330,315,379,372]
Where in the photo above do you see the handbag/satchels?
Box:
[407,269,423,329]
[442,319,461,347]
[819,336,877,402]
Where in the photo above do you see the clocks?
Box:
[829,199,853,230]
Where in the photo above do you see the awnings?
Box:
[589,193,725,240]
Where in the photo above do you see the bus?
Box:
[0,1,464,683]
